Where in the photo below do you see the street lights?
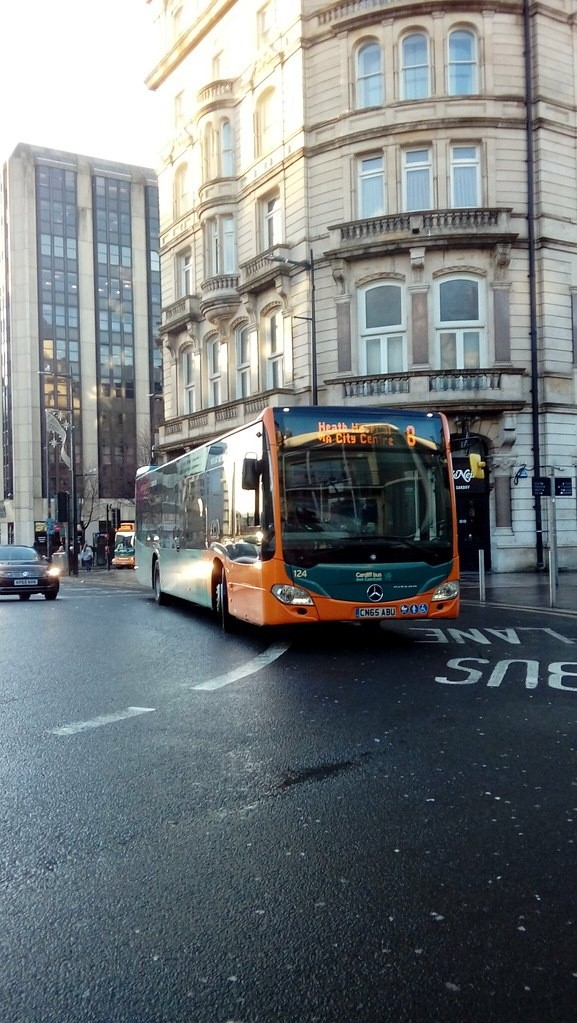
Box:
[37,367,80,576]
[264,249,320,407]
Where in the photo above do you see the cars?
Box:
[0,544,60,602]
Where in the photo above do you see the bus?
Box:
[131,402,488,631]
[112,523,138,570]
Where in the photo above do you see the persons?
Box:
[285,496,332,532]
[82,544,95,572]
[33,541,41,553]
